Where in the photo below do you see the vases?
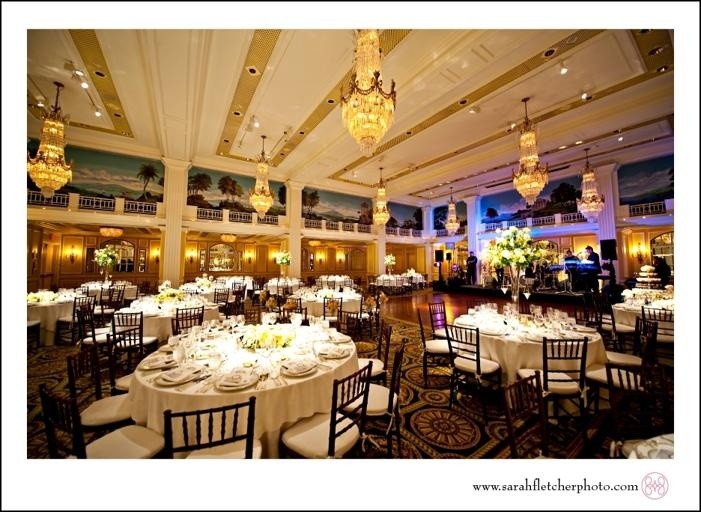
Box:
[508,265,523,313]
[388,265,393,276]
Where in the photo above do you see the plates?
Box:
[453,314,597,343]
[136,331,352,392]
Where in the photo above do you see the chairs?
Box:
[27,269,675,459]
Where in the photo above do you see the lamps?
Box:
[30,225,350,268]
[368,167,394,228]
[249,135,278,220]
[574,147,607,224]
[631,242,648,263]
[26,79,74,200]
[337,30,401,162]
[443,187,462,237]
[510,96,551,208]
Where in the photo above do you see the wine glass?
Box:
[165,313,328,364]
[179,282,224,292]
[37,287,84,302]
[295,285,319,299]
[468,302,577,334]
[85,279,132,288]
[131,293,204,312]
[622,292,652,308]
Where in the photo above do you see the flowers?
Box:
[383,253,397,267]
[478,224,560,269]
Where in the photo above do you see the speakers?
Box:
[446,253,451,260]
[435,250,443,261]
[600,239,618,261]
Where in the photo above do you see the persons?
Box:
[525,260,542,292]
[467,251,478,284]
[654,255,671,287]
[565,250,575,256]
[585,246,602,292]
